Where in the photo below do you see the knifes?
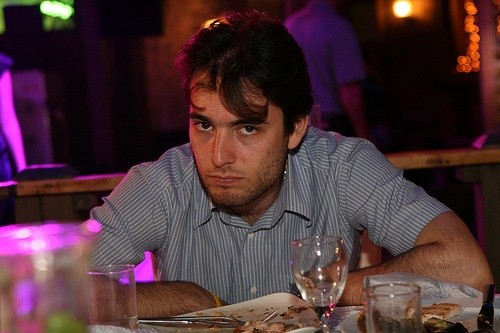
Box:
[476,284,496,333]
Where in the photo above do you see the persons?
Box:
[0,53,28,226]
[70,9,494,333]
[284,0,371,140]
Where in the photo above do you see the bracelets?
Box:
[208,288,221,308]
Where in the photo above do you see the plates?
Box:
[130,292,323,333]
[339,297,500,333]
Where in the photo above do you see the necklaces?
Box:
[283,159,288,182]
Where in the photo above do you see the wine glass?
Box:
[291,236,350,333]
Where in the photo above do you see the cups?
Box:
[8,246,90,333]
[366,282,423,333]
[87,264,139,330]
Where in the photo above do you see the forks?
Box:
[137,309,279,327]
